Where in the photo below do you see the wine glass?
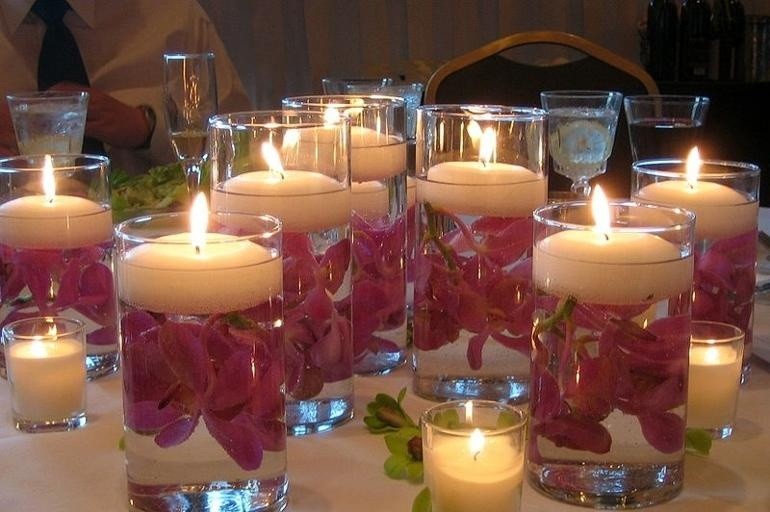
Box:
[160,51,219,213]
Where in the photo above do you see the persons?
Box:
[0,0,257,191]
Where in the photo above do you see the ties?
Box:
[29,0,113,197]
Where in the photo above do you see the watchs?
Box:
[125,104,158,152]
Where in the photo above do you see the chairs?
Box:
[423,32,662,105]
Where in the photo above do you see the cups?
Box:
[541,88,625,195]
[325,75,425,350]
[623,94,710,163]
[7,90,90,177]
